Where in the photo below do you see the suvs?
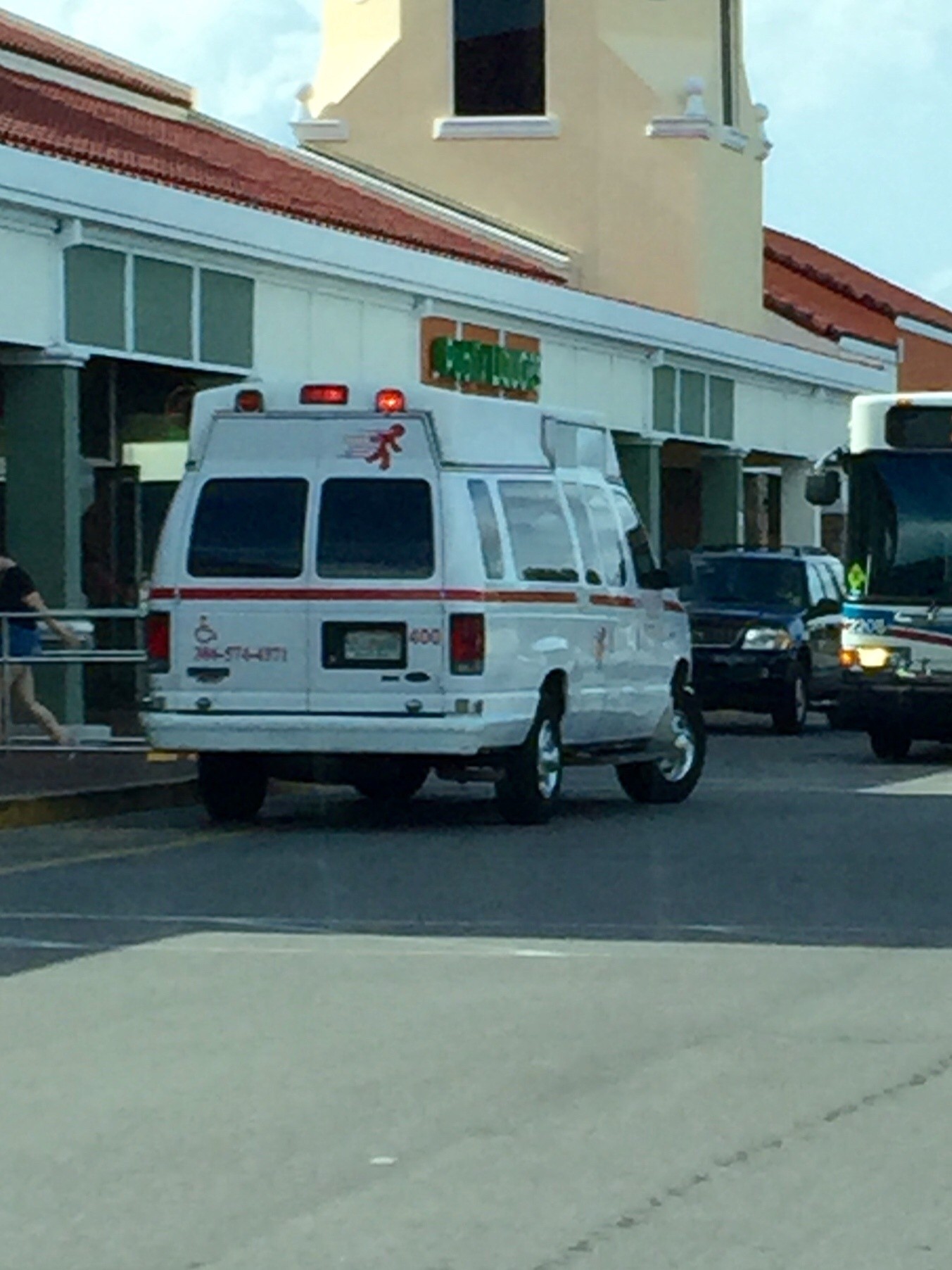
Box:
[670,544,847,736]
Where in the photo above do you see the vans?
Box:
[134,374,710,829]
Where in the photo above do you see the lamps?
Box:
[753,101,773,149]
[291,79,312,122]
[682,77,707,117]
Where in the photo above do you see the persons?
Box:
[0,551,81,746]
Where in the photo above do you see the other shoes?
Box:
[57,738,78,759]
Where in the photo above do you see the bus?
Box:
[805,389,952,767]
